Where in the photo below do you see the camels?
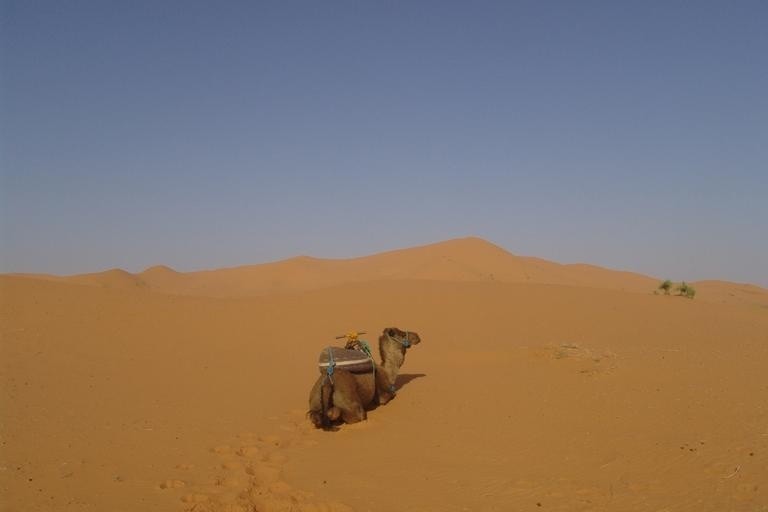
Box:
[306,328,421,432]
